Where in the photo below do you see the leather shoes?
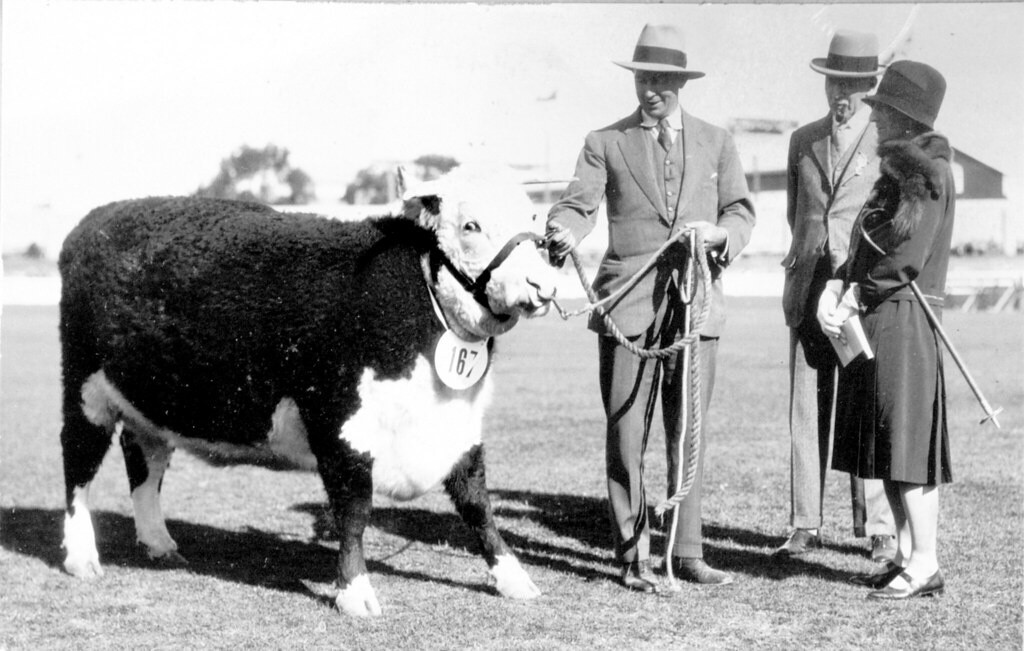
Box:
[870,533,898,563]
[775,528,822,555]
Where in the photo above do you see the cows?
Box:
[55,165,560,620]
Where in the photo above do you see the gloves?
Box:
[816,278,842,338]
[835,281,861,345]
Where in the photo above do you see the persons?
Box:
[830,59,952,598]
[546,20,755,593]
[778,29,901,562]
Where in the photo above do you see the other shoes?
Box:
[869,567,945,602]
[661,553,725,585]
[620,557,661,593]
[847,558,904,588]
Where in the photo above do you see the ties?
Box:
[658,119,672,152]
[832,124,848,153]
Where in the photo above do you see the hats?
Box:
[860,60,946,130]
[809,29,887,78]
[613,23,705,79]
